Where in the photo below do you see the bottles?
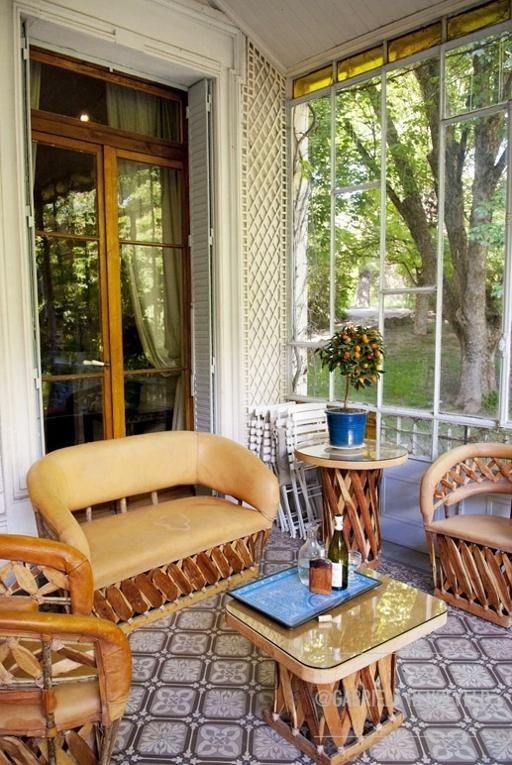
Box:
[297,513,349,595]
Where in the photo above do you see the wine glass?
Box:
[348,550,363,583]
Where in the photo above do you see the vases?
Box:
[327,408,367,448]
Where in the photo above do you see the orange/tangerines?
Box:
[336,324,387,387]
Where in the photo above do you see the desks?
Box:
[295,445,411,564]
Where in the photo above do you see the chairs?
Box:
[0,531,134,765]
[29,425,281,629]
[423,439,511,629]
